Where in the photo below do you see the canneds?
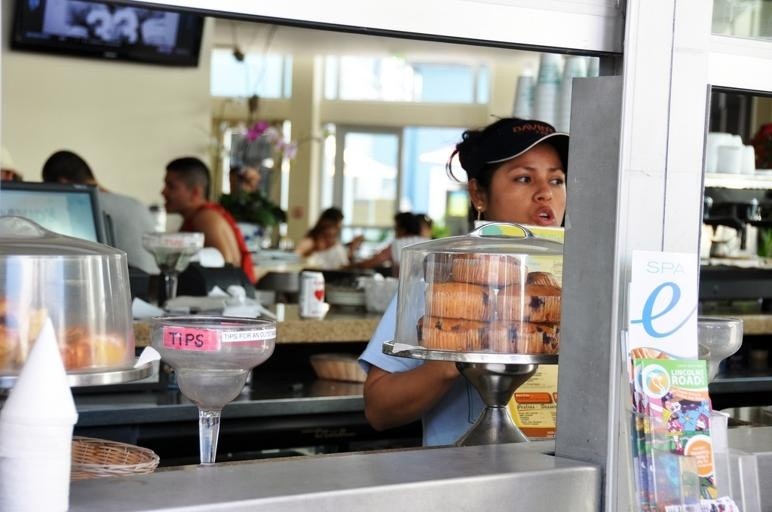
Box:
[298,271,325,320]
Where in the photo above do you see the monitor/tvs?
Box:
[0,181,104,284]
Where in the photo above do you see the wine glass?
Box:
[141,230,206,301]
[695,315,746,386]
[149,313,279,466]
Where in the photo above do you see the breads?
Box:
[525,272,558,288]
[423,253,452,282]
[419,316,487,350]
[496,283,563,323]
[425,280,495,322]
[490,323,561,355]
[451,253,527,288]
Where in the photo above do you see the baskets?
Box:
[310,354,370,383]
[70,436,159,484]
[310,381,368,398]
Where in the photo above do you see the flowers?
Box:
[218,118,297,204]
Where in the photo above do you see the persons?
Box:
[357,117,569,447]
[291,208,435,279]
[43,151,161,276]
[161,153,256,287]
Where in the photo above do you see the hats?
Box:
[473,116,570,164]
